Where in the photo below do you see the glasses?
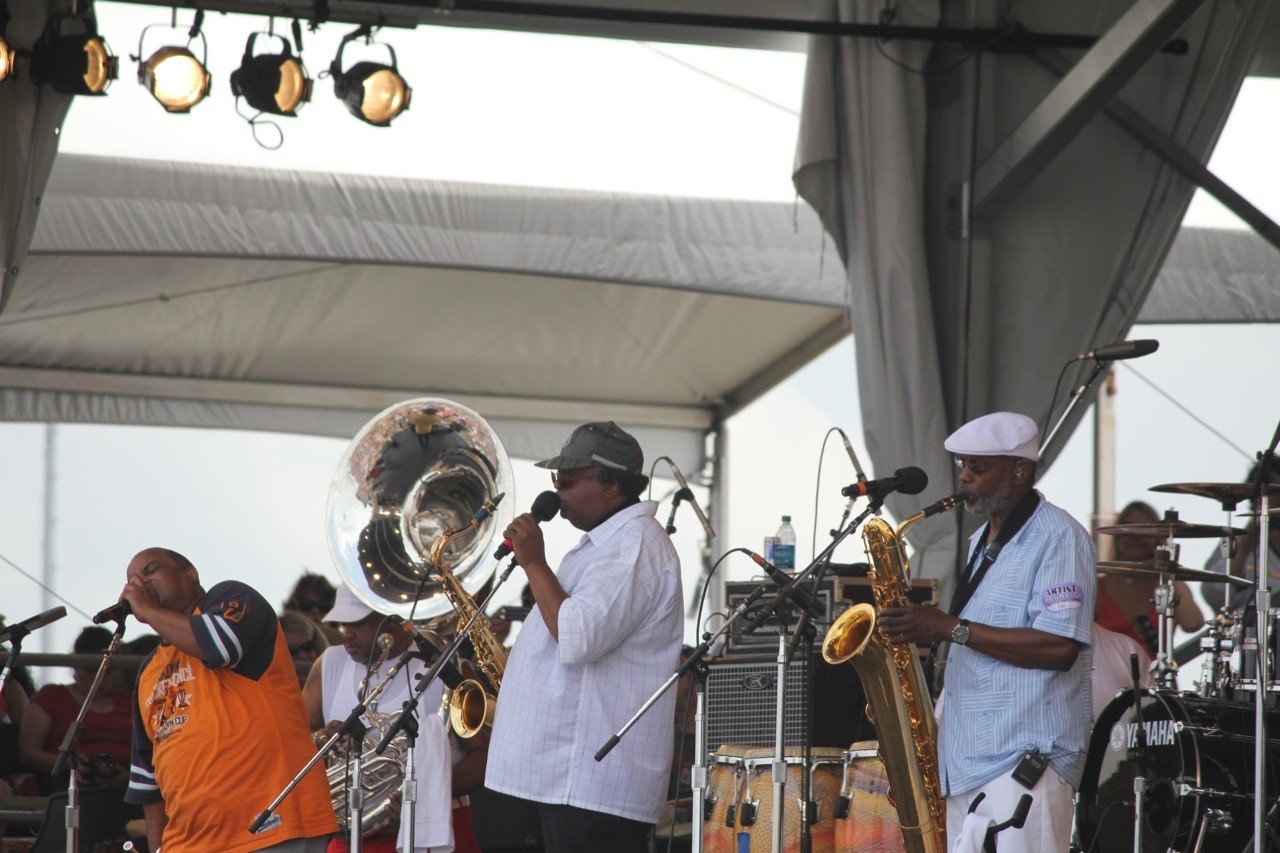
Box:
[954,457,1003,474]
[550,468,588,490]
[295,598,334,616]
[285,637,317,656]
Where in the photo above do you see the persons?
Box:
[302,581,489,853]
[877,412,1098,853]
[484,422,684,853]
[1093,501,1205,783]
[118,548,340,853]
[0,614,134,853]
[1201,456,1280,678]
[280,572,342,685]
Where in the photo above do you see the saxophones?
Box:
[822,492,970,852]
[429,493,507,741]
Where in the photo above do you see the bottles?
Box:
[773,516,796,580]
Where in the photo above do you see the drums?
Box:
[1071,685,1280,853]
[1222,603,1280,695]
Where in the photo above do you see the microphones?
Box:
[666,457,715,538]
[839,430,881,516]
[745,550,827,618]
[494,490,561,560]
[0,606,67,643]
[397,615,464,690]
[1123,801,1183,816]
[1072,339,1160,361]
[94,601,132,624]
[842,466,929,496]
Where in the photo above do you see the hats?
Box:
[534,420,646,477]
[943,412,1040,464]
[318,580,377,624]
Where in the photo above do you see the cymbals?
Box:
[1095,519,1251,538]
[1146,482,1280,517]
[1094,557,1256,588]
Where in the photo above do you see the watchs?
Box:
[952,619,970,646]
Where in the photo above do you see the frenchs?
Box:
[323,397,517,840]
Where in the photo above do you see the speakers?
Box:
[698,660,935,778]
